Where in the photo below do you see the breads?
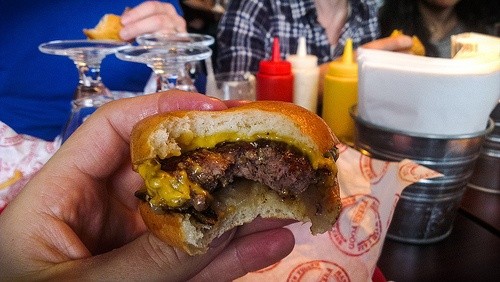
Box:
[83,14,136,42]
[389,29,425,56]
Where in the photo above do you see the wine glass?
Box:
[38,30,215,154]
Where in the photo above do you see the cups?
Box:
[215,71,257,100]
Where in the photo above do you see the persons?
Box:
[376,0,500,54]
[212,0,412,83]
[0,90,306,282]
[0,0,187,139]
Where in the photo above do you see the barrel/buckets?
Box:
[350,104,494,243]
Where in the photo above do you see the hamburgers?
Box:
[131,100,341,255]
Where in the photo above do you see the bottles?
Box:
[288,37,319,113]
[256,36,293,102]
[323,38,360,138]
